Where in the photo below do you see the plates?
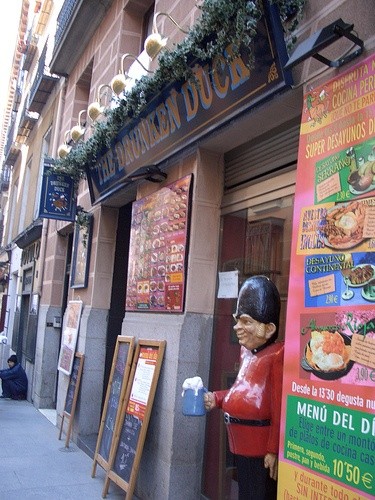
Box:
[361,287,375,301]
[348,183,375,195]
[344,264,375,287]
[317,204,364,249]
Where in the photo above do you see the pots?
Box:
[301,318,375,380]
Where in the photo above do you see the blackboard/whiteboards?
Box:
[104,338,167,493]
[62,352,85,420]
[94,335,134,473]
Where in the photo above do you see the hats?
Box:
[7,355,18,363]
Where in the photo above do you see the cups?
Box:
[181,376,208,416]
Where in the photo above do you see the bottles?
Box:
[346,147,356,172]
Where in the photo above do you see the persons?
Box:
[202,275,285,500]
[0,355,28,400]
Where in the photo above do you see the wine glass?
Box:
[341,254,354,300]
